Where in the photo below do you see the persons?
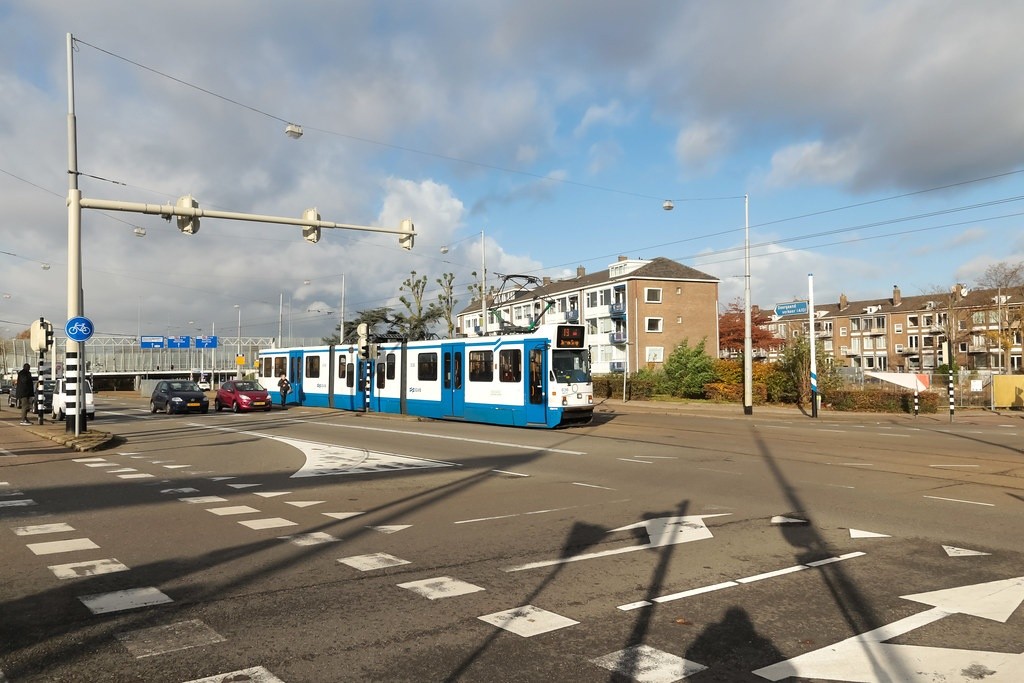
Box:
[278,373,291,410]
[418,353,576,404]
[15,363,35,425]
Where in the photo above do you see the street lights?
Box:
[662,193,754,415]
[441,230,488,334]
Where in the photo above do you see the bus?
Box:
[259,323,597,432]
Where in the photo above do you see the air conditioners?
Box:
[615,361,624,369]
[613,303,623,311]
[568,312,577,318]
[613,332,623,340]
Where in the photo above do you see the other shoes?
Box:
[20,420,33,425]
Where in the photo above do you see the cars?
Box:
[0,366,272,423]
